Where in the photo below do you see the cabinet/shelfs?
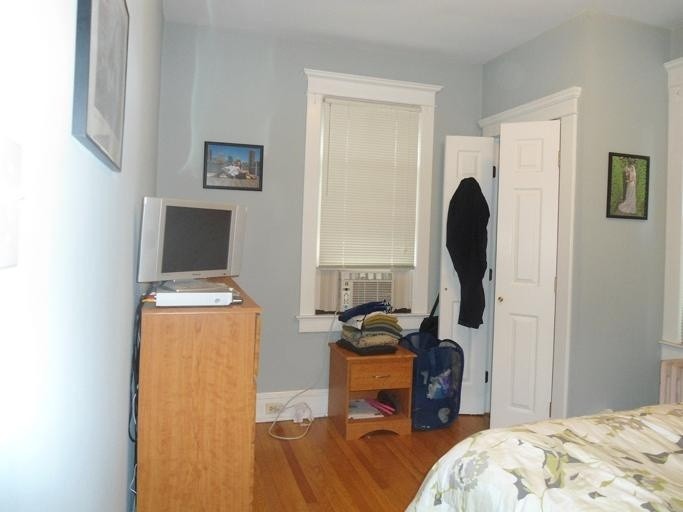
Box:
[132,275,260,511]
[326,341,416,442]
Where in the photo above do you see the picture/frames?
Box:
[605,149,650,221]
[70,0,132,174]
[202,140,265,192]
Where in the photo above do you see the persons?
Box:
[212,159,256,180]
[618,163,637,215]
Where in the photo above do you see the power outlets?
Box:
[265,401,282,412]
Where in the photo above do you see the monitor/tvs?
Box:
[137,197,239,290]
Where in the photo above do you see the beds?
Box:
[404,397,683,512]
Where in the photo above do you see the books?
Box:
[349,397,384,419]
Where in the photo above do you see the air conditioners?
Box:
[335,269,395,315]
